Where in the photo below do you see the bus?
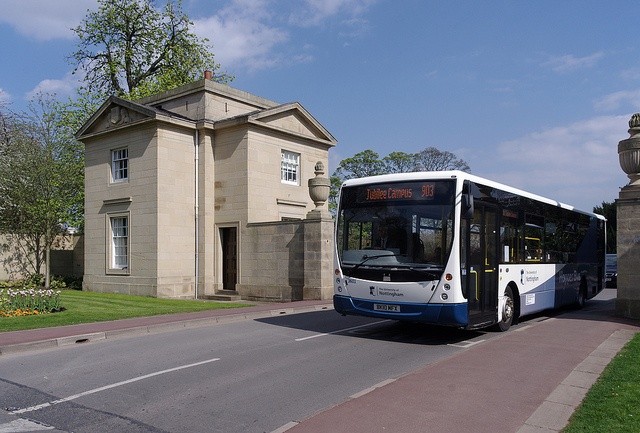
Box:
[333,170,608,330]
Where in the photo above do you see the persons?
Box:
[428,246,442,263]
[369,223,394,249]
